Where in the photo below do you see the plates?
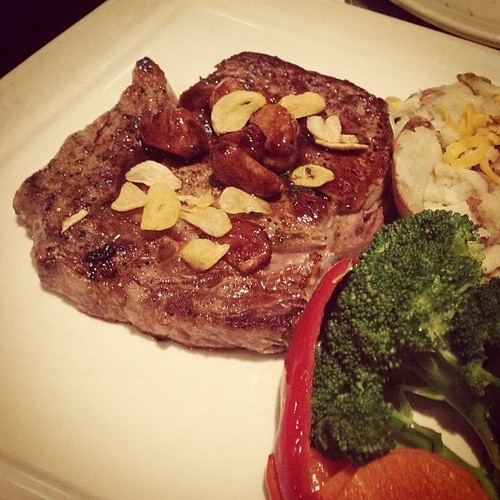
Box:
[0,0,498,500]
[394,0,500,51]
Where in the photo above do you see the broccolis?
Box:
[308,208,500,500]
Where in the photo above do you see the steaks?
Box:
[12,51,395,353]
